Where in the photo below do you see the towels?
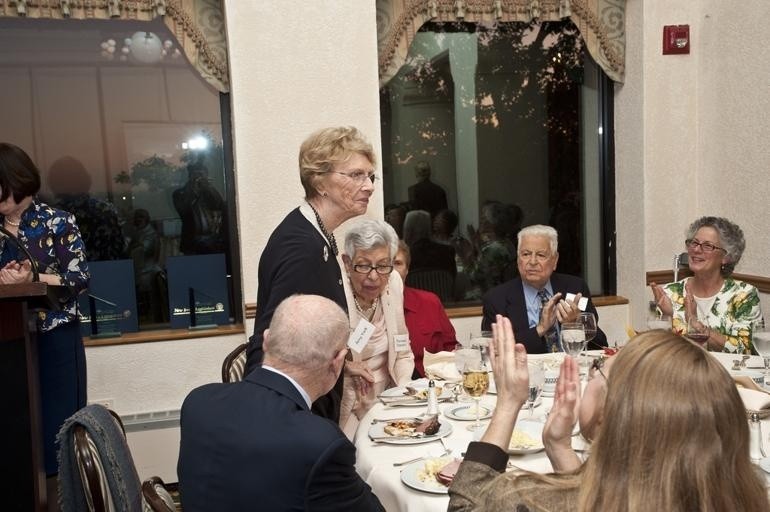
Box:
[421,348,463,383]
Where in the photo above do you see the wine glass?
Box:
[575,312,597,360]
[528,359,545,423]
[469,330,493,360]
[461,359,489,430]
[454,346,482,379]
[686,316,710,346]
[752,320,770,383]
[560,322,586,357]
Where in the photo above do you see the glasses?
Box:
[585,356,608,383]
[685,238,726,252]
[353,264,393,275]
[330,168,378,182]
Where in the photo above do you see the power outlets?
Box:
[88,399,113,410]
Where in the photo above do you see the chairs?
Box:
[142,476,181,512]
[406,267,454,302]
[222,343,247,383]
[72,409,141,512]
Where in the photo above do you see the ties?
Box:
[539,291,559,353]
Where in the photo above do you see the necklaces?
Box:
[304,196,338,256]
[5,216,21,227]
[354,296,378,313]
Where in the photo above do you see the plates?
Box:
[444,402,495,421]
[368,415,454,445]
[508,420,545,454]
[379,383,453,405]
[400,459,458,494]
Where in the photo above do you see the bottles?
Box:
[426,379,441,415]
[747,412,766,460]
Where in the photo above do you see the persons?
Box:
[393,240,464,381]
[389,204,405,238]
[130,210,163,289]
[177,293,387,512]
[646,215,765,356]
[465,204,524,302]
[173,161,227,257]
[447,314,770,512]
[339,216,415,432]
[402,211,459,294]
[546,199,583,274]
[45,155,126,264]
[433,210,460,267]
[242,126,380,427]
[0,142,91,512]
[407,159,448,230]
[481,225,609,354]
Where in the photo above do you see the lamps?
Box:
[100,30,180,64]
